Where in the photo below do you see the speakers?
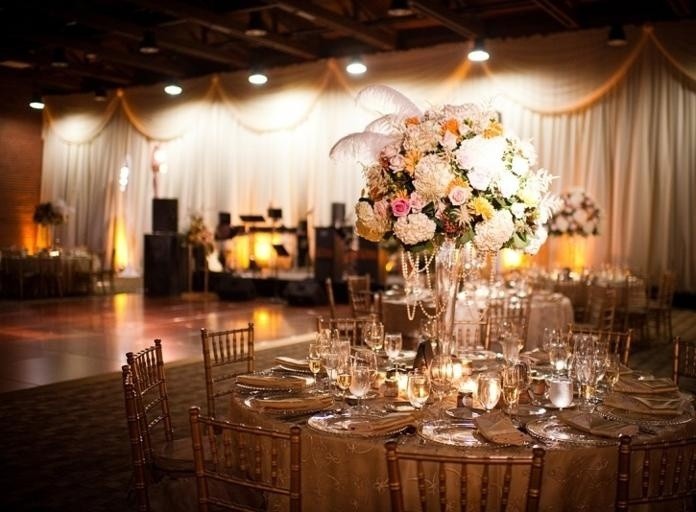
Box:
[144,234,190,297]
[315,225,353,280]
[151,198,178,236]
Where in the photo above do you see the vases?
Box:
[429,234,466,369]
[52,226,59,250]
[568,235,587,279]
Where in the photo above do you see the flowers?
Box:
[32,201,76,228]
[548,194,602,235]
[353,106,559,254]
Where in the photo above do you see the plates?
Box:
[235,349,692,450]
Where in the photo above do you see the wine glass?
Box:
[307,320,621,417]
[580,260,639,279]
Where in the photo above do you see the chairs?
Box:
[0,243,117,300]
[384,440,546,512]
[126,339,225,484]
[616,433,696,512]
[188,405,301,512]
[200,322,255,435]
[672,335,696,397]
[122,365,266,512]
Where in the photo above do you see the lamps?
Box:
[30,90,46,109]
[467,31,490,63]
[164,71,184,98]
[347,46,367,76]
[248,61,269,84]
